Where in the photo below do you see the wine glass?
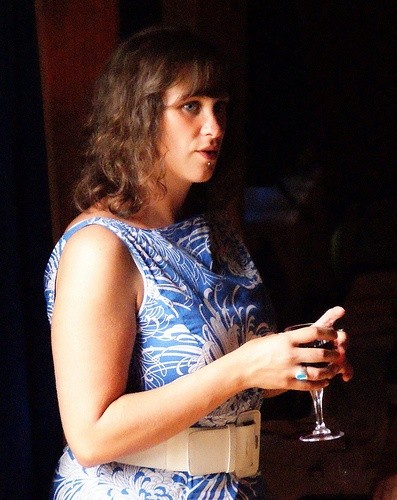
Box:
[284,320,346,442]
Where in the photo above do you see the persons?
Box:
[42,28,357,500]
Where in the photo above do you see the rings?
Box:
[292,368,312,384]
